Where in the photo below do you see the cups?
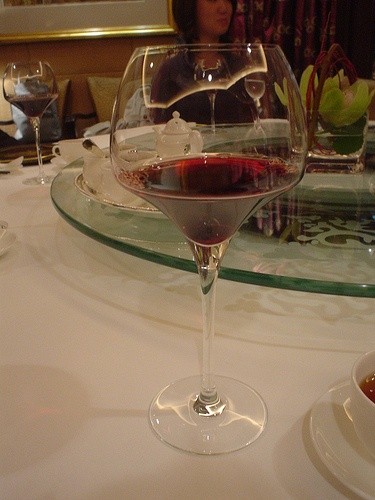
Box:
[351,350,375,458]
[52,139,83,162]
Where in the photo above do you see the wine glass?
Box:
[2,60,59,186]
[109,43,311,455]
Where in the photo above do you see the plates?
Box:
[0,232,16,255]
[74,173,169,219]
[0,143,59,164]
[306,150,360,161]
[309,379,375,500]
[50,157,69,168]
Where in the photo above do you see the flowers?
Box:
[274,65,375,128]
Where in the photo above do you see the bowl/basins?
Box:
[254,117,289,139]
[83,144,156,205]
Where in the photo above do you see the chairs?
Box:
[1,74,152,153]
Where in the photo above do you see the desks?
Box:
[1,124,375,499]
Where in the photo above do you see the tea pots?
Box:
[152,111,203,158]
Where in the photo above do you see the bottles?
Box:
[365,125,375,168]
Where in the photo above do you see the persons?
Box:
[150,0,271,126]
